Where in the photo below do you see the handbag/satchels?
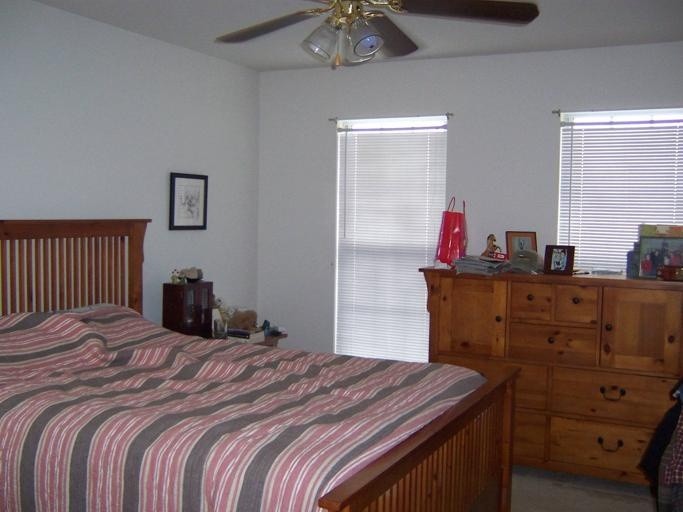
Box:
[436,210,469,267]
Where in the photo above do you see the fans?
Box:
[214,1,539,69]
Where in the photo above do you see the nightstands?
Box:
[186,326,288,349]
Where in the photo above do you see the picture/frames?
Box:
[544,245,575,275]
[505,230,538,262]
[637,236,683,280]
[168,171,208,230]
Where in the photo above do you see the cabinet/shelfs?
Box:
[510,281,549,318]
[444,354,547,405]
[513,409,550,461]
[600,286,681,374]
[556,283,598,325]
[546,416,655,475]
[509,324,596,365]
[162,280,213,339]
[553,369,677,427]
[439,277,506,357]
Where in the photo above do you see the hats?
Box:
[505,249,545,273]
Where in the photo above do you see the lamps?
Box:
[299,0,385,69]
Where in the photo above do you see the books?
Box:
[227,326,265,344]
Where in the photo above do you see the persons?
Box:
[640,238,683,277]
[552,249,567,270]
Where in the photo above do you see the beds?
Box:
[3,218,517,512]
